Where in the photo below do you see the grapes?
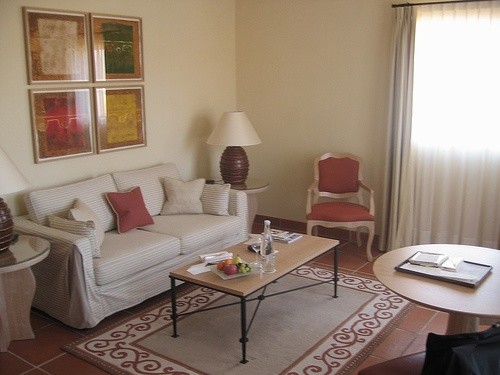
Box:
[237,263,253,274]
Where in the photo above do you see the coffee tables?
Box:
[167,230,341,364]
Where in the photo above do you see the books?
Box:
[262,228,302,243]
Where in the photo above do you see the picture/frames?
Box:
[95,85,147,154]
[22,5,92,85]
[27,88,95,163]
[88,11,144,83]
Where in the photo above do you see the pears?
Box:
[232,254,242,264]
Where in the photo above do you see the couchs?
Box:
[12,162,248,329]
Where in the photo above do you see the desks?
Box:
[373,243,500,333]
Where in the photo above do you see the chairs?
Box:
[304,151,379,262]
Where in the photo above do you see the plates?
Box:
[207,265,255,280]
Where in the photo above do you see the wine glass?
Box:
[251,238,261,261]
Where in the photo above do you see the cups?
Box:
[251,256,263,274]
[263,254,276,273]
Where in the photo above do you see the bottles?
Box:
[260,220,273,255]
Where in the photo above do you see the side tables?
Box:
[215,176,271,233]
[0,234,50,353]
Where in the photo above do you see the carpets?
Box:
[61,262,413,375]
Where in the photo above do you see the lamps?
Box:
[206,110,262,183]
[0,145,30,253]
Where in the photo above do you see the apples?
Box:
[218,259,238,275]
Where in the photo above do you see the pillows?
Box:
[161,176,205,217]
[47,213,102,258]
[67,199,105,246]
[201,183,231,216]
[104,186,154,232]
